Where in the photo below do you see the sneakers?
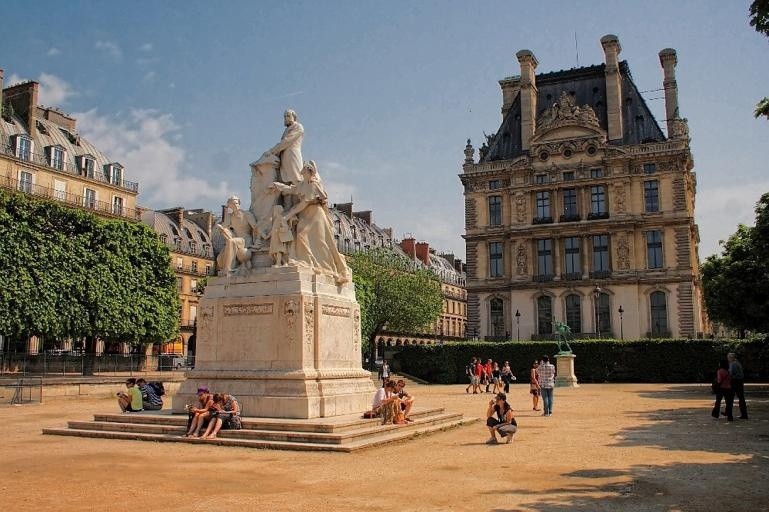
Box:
[506,434,514,444]
[711,411,750,422]
[486,437,498,444]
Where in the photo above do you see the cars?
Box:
[47,350,186,370]
[375,356,383,365]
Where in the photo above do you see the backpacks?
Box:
[148,381,165,396]
[465,365,471,377]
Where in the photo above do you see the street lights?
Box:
[594,281,603,338]
[618,305,624,339]
[515,307,521,341]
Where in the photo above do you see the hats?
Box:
[495,392,506,401]
[196,386,208,396]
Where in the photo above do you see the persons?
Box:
[270,106,306,212]
[485,391,517,444]
[117,376,142,412]
[369,380,399,424]
[530,359,545,412]
[465,355,516,394]
[266,160,348,283]
[394,380,415,424]
[377,359,391,388]
[264,201,316,269]
[537,355,556,417]
[723,352,751,419]
[135,378,163,410]
[709,357,735,421]
[201,393,243,439]
[185,384,222,437]
[213,192,265,273]
